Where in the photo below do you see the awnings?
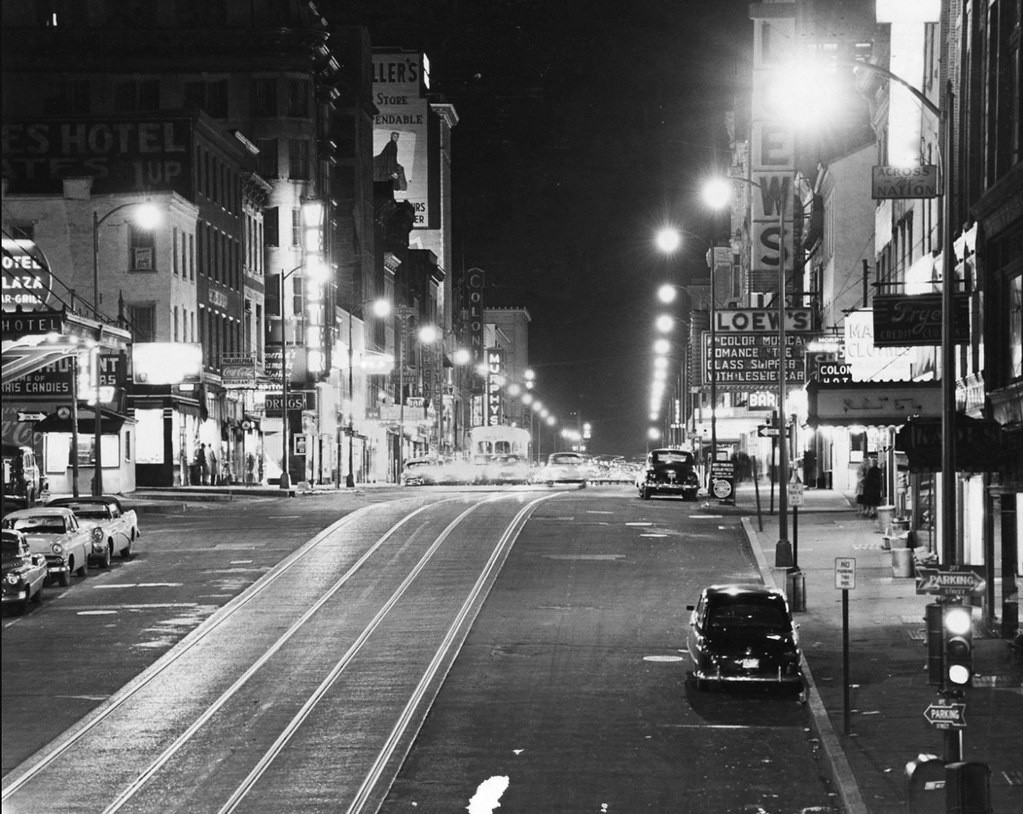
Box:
[33,404,139,436]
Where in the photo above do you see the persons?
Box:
[855,456,882,518]
[198,443,217,486]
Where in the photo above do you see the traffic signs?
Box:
[915,560,988,597]
[923,703,967,726]
[16,411,48,422]
[757,425,791,438]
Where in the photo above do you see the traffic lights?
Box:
[942,603,973,690]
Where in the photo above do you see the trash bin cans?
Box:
[905,754,945,814]
[891,549,911,577]
[944,761,991,814]
[877,505,894,533]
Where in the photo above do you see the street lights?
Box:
[93,197,157,497]
[705,172,795,567]
[778,47,964,763]
[281,259,390,489]
[649,224,714,460]
[399,322,554,483]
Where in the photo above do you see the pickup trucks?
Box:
[49,496,140,569]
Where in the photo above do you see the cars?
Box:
[1,528,47,609]
[472,453,545,485]
[638,448,701,500]
[686,585,801,691]
[546,451,638,488]
[401,457,470,486]
[6,506,93,588]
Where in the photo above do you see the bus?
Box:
[469,425,530,458]
